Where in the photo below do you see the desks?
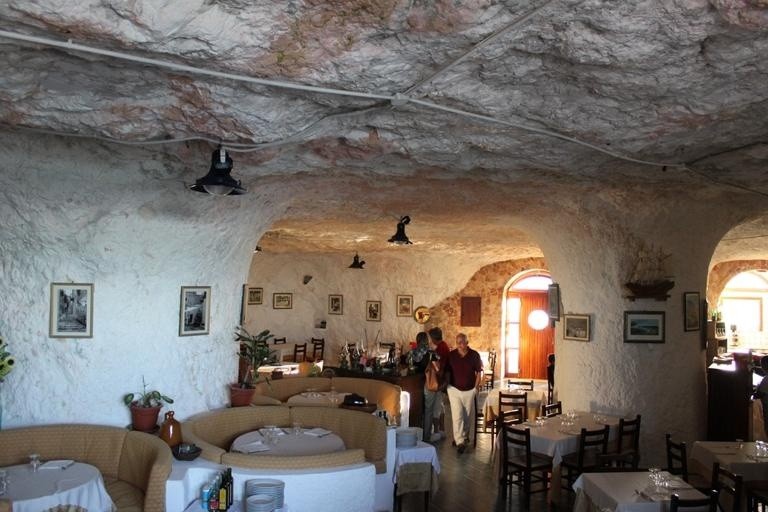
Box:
[1,460,100,510]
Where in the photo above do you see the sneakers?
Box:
[458,444,465,455]
[452,439,469,447]
[430,430,447,444]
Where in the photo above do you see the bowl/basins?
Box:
[171,443,202,461]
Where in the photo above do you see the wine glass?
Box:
[646,466,674,499]
[260,419,305,446]
[754,440,768,457]
[531,409,609,432]
[28,453,41,473]
[304,385,340,406]
[0,468,10,495]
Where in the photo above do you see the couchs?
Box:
[0,423,172,510]
[181,376,411,512]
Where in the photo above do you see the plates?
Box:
[244,478,285,511]
[396,426,424,449]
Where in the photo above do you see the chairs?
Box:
[254,336,325,362]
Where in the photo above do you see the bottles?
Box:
[159,410,183,449]
[201,466,234,512]
[710,304,721,322]
[378,410,397,429]
[374,359,382,374]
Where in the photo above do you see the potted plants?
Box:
[120,374,175,429]
[226,325,276,405]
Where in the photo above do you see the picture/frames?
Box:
[396,294,414,317]
[48,280,96,340]
[548,284,700,344]
[366,300,382,322]
[248,287,294,310]
[328,294,343,316]
[177,284,213,335]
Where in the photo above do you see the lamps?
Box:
[187,136,250,199]
[349,251,368,270]
[387,216,413,245]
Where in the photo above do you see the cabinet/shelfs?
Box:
[706,319,728,371]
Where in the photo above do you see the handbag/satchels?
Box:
[425,361,443,391]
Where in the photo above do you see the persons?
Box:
[443,333,483,453]
[752,354,768,435]
[429,326,450,441]
[546,353,556,383]
[396,331,438,442]
